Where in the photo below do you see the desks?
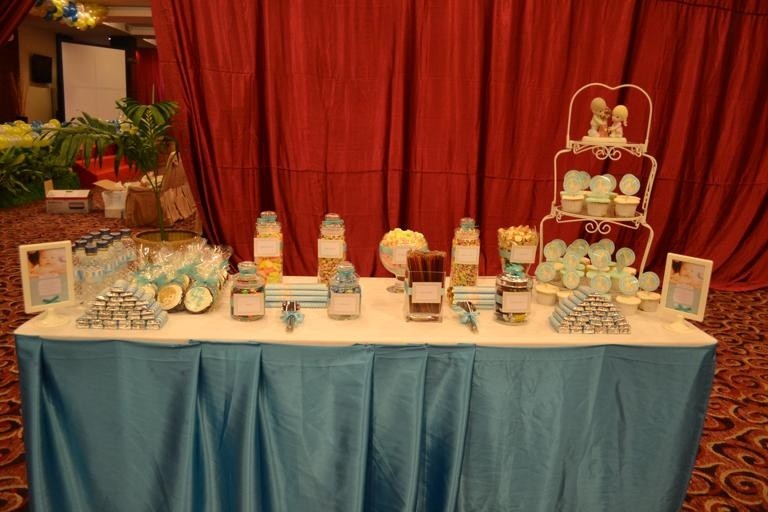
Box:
[11,270,727,509]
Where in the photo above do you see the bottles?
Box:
[315,212,347,285]
[32,119,43,130]
[448,217,481,288]
[228,261,266,322]
[112,120,120,133]
[492,264,533,327]
[326,260,362,322]
[70,227,139,310]
[251,209,284,286]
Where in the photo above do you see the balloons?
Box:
[29,0,110,32]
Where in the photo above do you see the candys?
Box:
[380,227,428,278]
[310,212,350,279]
[449,216,480,286]
[228,261,267,321]
[255,210,283,284]
[326,264,363,320]
[496,225,539,274]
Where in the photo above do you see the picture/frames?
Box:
[656,251,716,324]
[18,238,80,317]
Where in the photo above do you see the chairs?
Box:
[123,150,199,228]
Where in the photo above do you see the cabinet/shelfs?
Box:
[534,82,660,308]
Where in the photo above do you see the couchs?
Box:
[71,144,138,191]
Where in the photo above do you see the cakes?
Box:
[533,238,661,317]
[558,168,641,218]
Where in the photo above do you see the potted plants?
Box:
[106,96,204,256]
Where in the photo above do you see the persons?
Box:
[30,250,67,273]
[669,262,706,286]
[581,97,629,140]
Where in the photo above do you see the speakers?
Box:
[111,35,136,48]
[32,54,52,84]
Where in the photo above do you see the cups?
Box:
[533,255,661,316]
[558,188,641,219]
[498,230,539,277]
[403,270,446,325]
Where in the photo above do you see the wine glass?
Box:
[378,242,427,296]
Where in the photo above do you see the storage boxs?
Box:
[40,178,94,217]
[92,177,130,223]
